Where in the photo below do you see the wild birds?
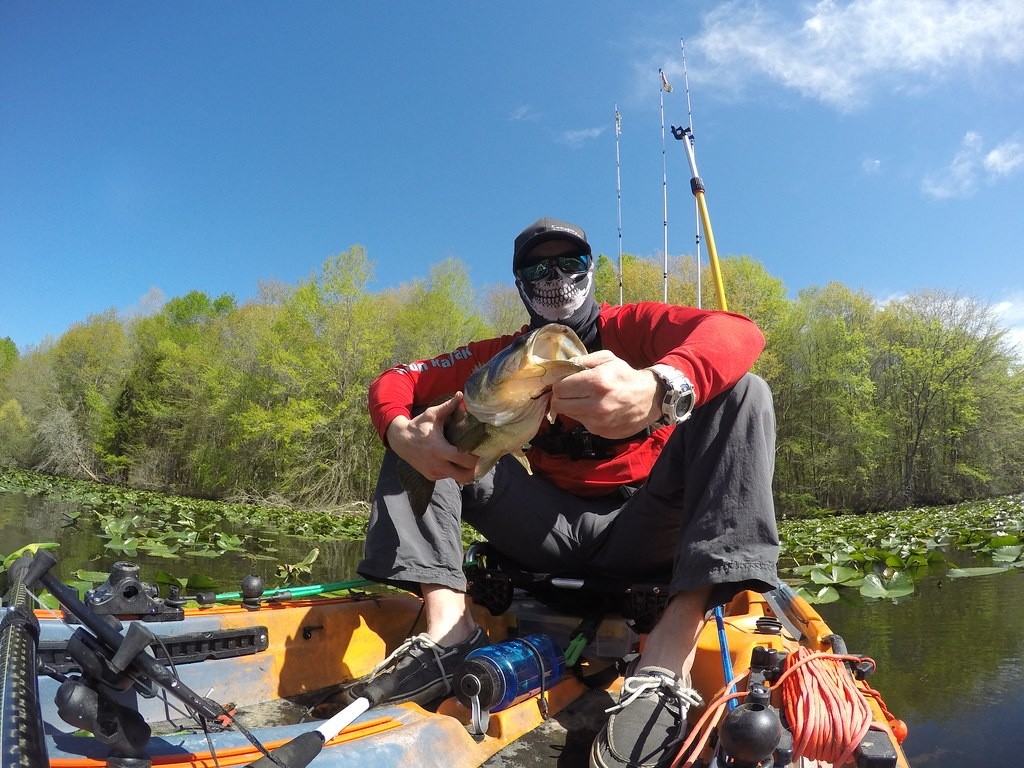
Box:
[441,321,594,491]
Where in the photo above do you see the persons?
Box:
[344,217,781,768]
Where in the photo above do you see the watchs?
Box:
[647,363,697,427]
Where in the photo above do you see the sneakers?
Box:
[588,654,705,768]
[346,623,496,709]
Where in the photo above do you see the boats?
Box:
[0,572,910,768]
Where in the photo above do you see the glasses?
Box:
[515,251,591,283]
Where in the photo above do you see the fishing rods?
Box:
[658,66,670,301]
[677,36,703,309]
[613,101,626,304]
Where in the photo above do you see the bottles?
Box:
[453,632,565,736]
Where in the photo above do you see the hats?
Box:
[513,216,592,274]
